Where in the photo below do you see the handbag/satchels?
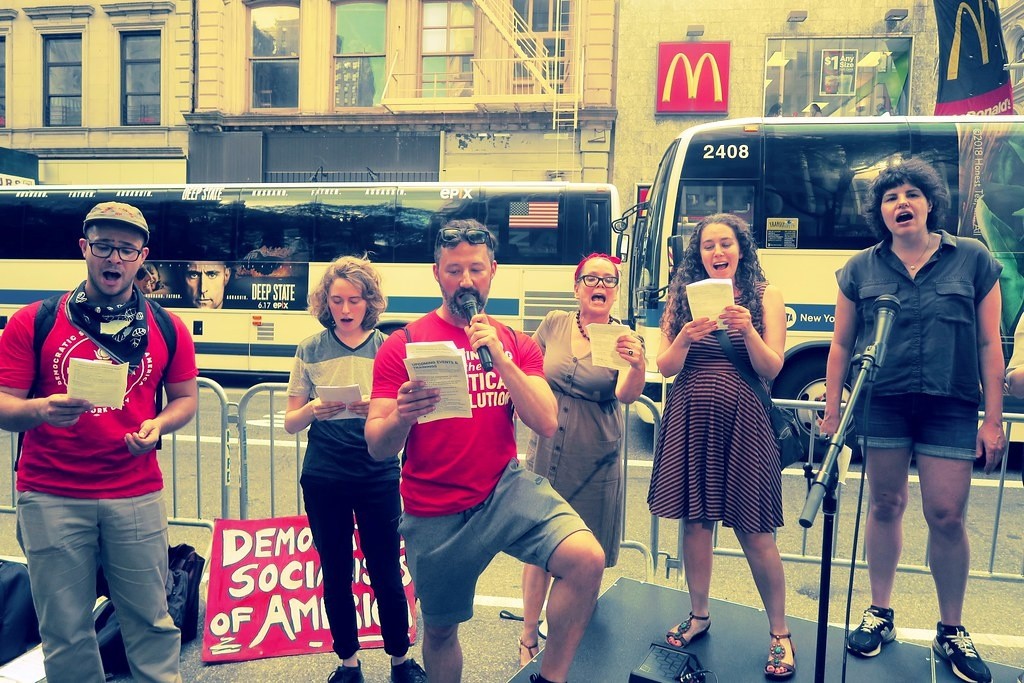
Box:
[166,544,205,643]
[767,406,805,471]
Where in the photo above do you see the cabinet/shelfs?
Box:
[258,89,272,108]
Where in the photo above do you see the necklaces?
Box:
[576,310,613,341]
[904,234,931,270]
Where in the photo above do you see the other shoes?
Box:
[327,659,364,683]
[390,658,426,683]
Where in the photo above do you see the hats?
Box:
[82,201,149,246]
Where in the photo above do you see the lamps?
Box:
[687,25,704,37]
[309,166,327,182]
[787,10,807,22]
[884,8,908,21]
[366,166,377,180]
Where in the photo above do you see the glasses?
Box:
[576,275,619,289]
[437,228,495,251]
[85,236,142,262]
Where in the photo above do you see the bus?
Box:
[609,115,1024,465]
[0,182,623,378]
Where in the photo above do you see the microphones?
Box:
[866,294,902,383]
[461,293,495,372]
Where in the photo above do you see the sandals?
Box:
[763,631,796,681]
[664,611,711,649]
[519,639,540,669]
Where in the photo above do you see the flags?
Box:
[933,0,1013,116]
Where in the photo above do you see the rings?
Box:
[629,349,633,355]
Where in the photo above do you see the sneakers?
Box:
[932,622,993,683]
[847,605,896,659]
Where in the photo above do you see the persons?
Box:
[821,158,1008,683]
[1005,312,1024,487]
[519,254,645,671]
[284,255,428,683]
[183,262,258,309]
[134,263,169,298]
[657,214,797,678]
[0,202,199,683]
[364,217,606,683]
